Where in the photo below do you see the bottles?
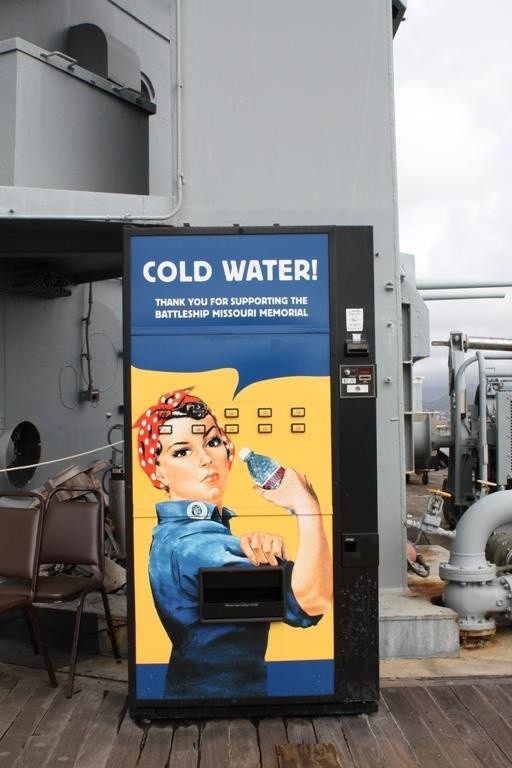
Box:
[236,447,297,517]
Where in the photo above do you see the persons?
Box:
[130,385,335,701]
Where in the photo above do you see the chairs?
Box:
[24,486,121,699]
[0,490,58,689]
